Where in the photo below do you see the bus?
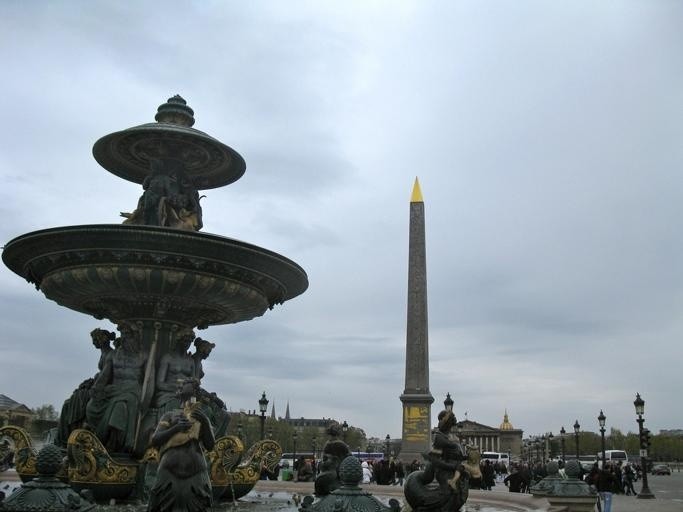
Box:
[278,453,317,468]
[352,452,385,463]
[597,450,628,474]
[480,451,510,468]
[552,454,597,470]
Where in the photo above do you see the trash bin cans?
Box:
[281,470,288,481]
[603,493,612,512]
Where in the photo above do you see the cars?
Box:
[651,464,670,475]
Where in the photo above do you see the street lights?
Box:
[508,445,511,465]
[560,426,566,463]
[597,409,607,470]
[292,431,298,469]
[634,392,656,498]
[259,391,269,440]
[520,432,554,465]
[574,420,580,460]
[238,419,242,439]
[312,435,316,467]
[386,434,390,460]
[342,421,348,441]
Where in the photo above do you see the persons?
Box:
[180,174,209,232]
[154,322,214,432]
[146,375,217,511]
[191,334,230,438]
[296,449,641,511]
[133,142,179,225]
[433,409,471,462]
[82,323,157,455]
[50,326,115,446]
[320,422,349,463]
